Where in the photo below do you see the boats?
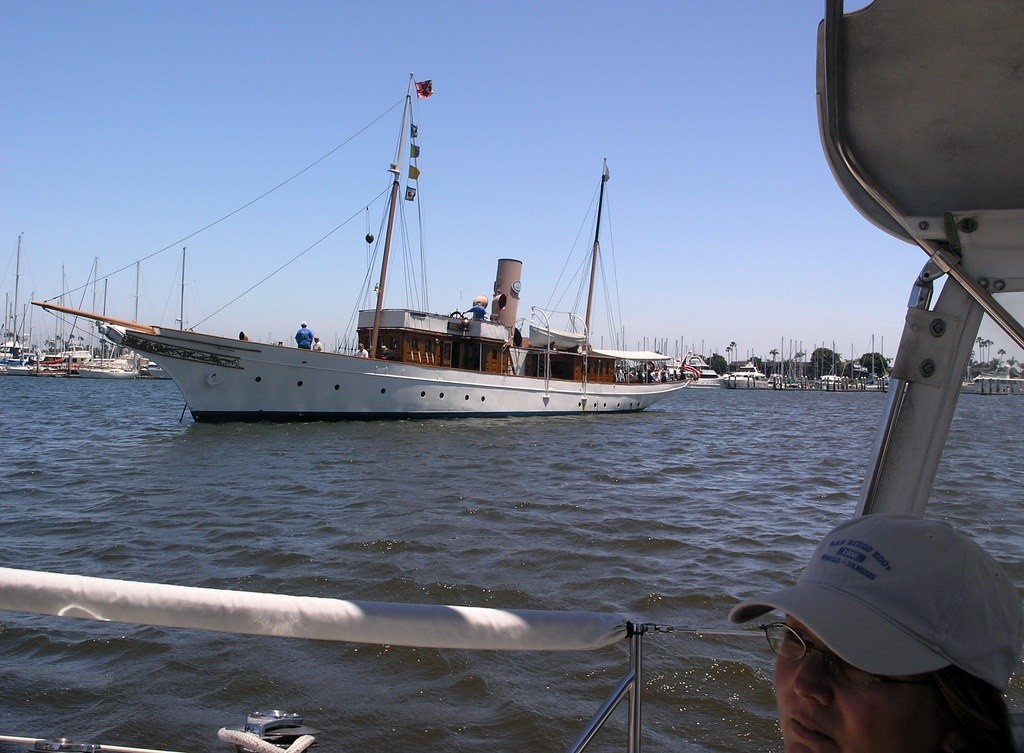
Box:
[29,72,691,424]
[819,375,850,390]
[666,351,723,388]
[720,361,773,389]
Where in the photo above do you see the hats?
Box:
[301,322,307,326]
[727,513,1020,693]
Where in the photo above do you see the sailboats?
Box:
[0,232,194,380]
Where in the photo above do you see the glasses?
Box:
[757,621,918,689]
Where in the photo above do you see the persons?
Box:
[728,513,1024,753]
[637,365,686,383]
[355,343,369,358]
[312,336,323,351]
[462,300,486,321]
[295,322,313,349]
[381,346,395,360]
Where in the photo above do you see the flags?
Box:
[683,364,702,378]
